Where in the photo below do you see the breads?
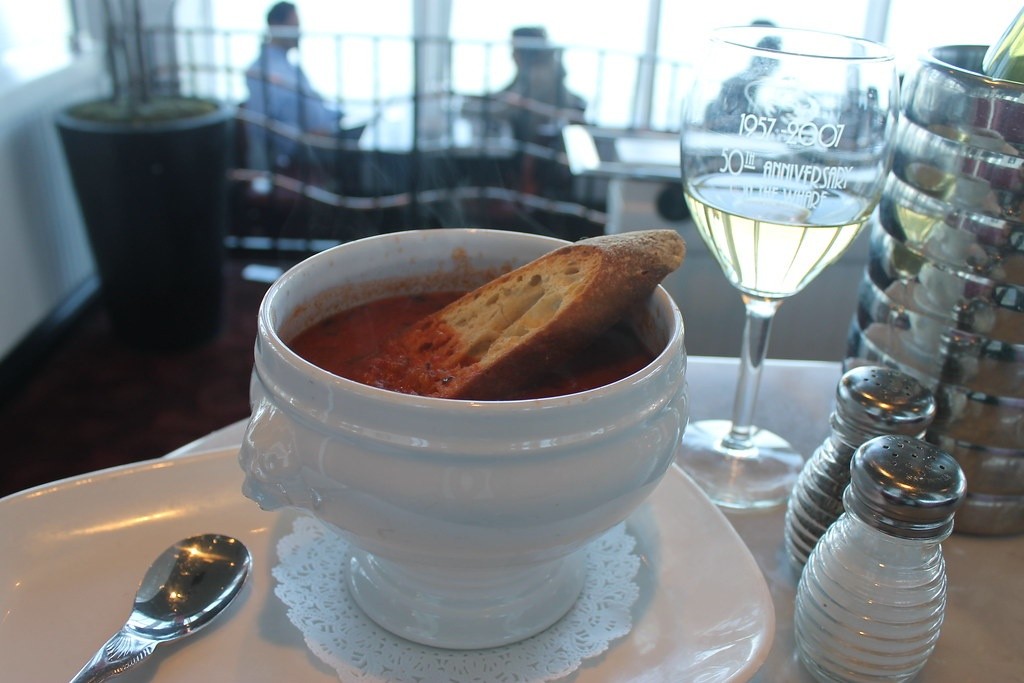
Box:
[406,229,686,400]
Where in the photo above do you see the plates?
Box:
[0,450,777,683]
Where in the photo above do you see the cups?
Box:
[853,47,1023,539]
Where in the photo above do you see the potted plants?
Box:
[49,0,241,356]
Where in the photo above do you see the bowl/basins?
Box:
[240,229,696,651]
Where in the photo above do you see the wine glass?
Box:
[676,28,903,512]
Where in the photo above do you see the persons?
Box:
[705,37,794,135]
[245,2,359,170]
[482,27,587,154]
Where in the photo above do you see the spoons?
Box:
[67,534,253,683]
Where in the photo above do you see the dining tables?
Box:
[161,350,1020,683]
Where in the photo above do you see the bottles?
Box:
[784,364,938,577]
[792,434,967,682]
